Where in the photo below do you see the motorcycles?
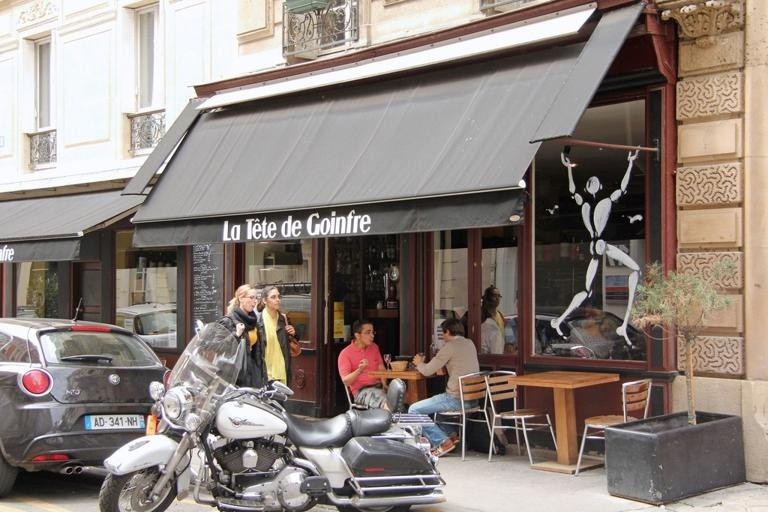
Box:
[98,319,446,512]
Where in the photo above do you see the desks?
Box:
[368,367,447,411]
[506,369,619,474]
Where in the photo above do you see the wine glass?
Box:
[383,353,392,372]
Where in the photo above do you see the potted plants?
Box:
[592,256,747,506]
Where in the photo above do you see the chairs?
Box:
[433,371,497,460]
[574,378,653,478]
[343,379,369,411]
[481,371,558,466]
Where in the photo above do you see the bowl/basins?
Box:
[390,360,409,374]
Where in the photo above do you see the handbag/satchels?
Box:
[212,339,248,379]
[466,414,504,454]
[288,336,302,357]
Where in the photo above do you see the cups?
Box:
[418,352,426,364]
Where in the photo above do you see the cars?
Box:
[503,309,646,360]
[0,317,172,497]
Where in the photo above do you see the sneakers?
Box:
[430,435,460,456]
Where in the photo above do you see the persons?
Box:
[337,318,395,415]
[256,285,301,411]
[481,284,506,355]
[408,318,481,458]
[211,283,268,389]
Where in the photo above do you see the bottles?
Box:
[376,300,383,311]
[429,334,439,360]
[557,235,586,260]
